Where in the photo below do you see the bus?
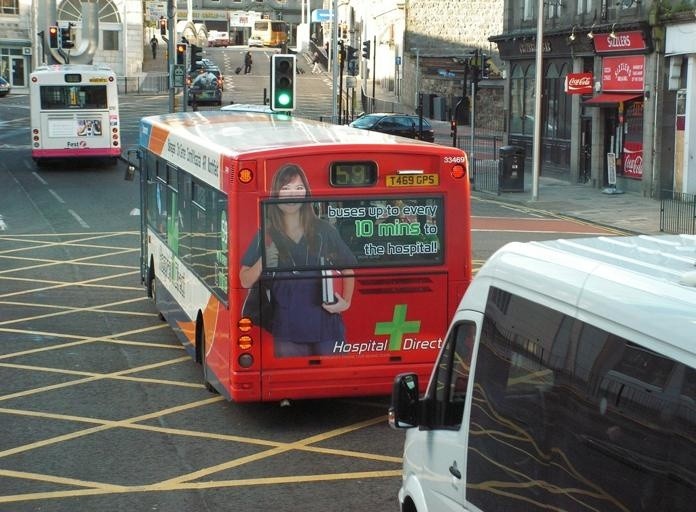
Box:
[251,20,289,47]
[29,61,120,168]
[137,105,471,408]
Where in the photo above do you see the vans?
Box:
[385,230,696,512]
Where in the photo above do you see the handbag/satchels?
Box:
[242,270,275,332]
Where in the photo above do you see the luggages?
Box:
[235,63,245,74]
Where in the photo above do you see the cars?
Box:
[0,77,10,98]
[185,58,223,107]
[247,36,264,48]
[516,114,553,134]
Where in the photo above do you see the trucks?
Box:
[205,29,229,49]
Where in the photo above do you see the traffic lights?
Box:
[159,19,166,36]
[191,44,202,72]
[272,54,296,111]
[60,28,72,48]
[348,47,359,61]
[176,43,187,65]
[362,40,371,59]
[49,26,59,49]
[450,120,459,130]
[482,54,491,80]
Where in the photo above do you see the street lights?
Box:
[408,47,425,108]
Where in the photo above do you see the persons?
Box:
[287,46,300,74]
[310,51,323,75]
[180,35,189,45]
[243,50,254,75]
[150,33,158,60]
[238,163,355,357]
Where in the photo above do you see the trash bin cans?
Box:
[497,146,525,192]
[423,94,446,120]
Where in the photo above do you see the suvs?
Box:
[346,111,436,143]
